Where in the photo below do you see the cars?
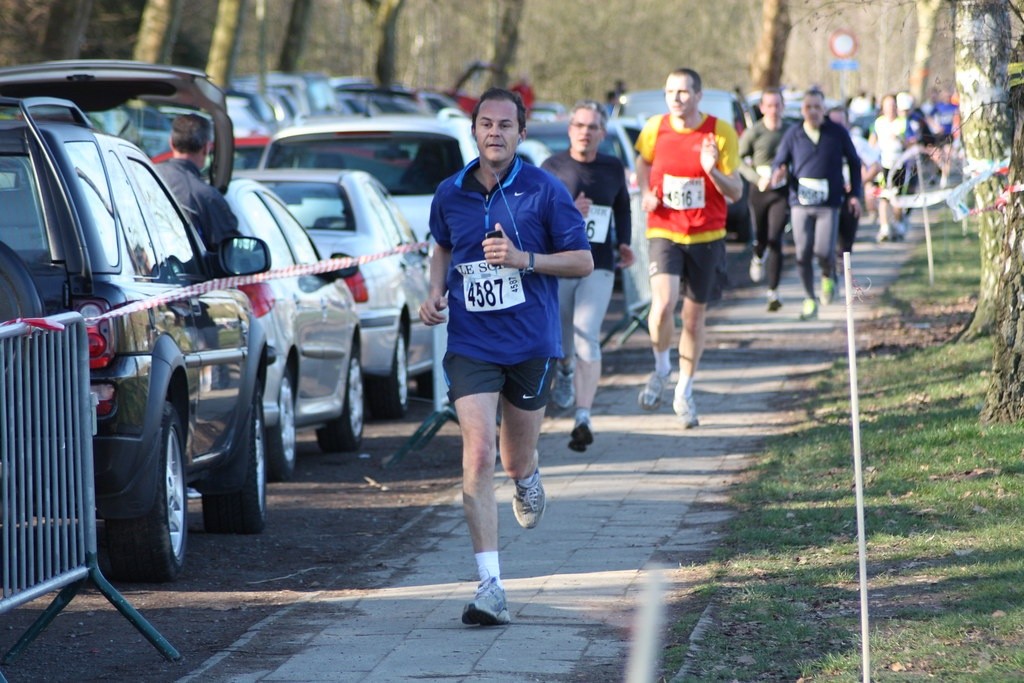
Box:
[1,57,839,247]
[226,168,436,423]
[201,175,365,481]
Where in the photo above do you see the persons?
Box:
[634,68,743,429]
[419,87,594,626]
[739,88,794,312]
[772,89,861,321]
[603,80,627,117]
[541,101,635,445]
[510,71,535,118]
[154,114,244,278]
[826,91,934,275]
[920,87,968,190]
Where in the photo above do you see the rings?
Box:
[494,252,496,258]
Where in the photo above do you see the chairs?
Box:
[314,152,344,169]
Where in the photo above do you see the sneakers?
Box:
[552,362,574,408]
[673,391,699,427]
[638,370,671,410]
[568,422,594,452]
[462,577,510,625]
[513,468,545,528]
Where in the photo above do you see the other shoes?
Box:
[800,297,818,320]
[749,245,770,283]
[767,295,781,312]
[877,224,893,243]
[819,276,834,306]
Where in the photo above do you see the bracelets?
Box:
[523,252,535,274]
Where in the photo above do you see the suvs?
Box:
[259,105,482,260]
[612,90,758,246]
[0,92,272,582]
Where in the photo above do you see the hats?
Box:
[897,92,914,110]
